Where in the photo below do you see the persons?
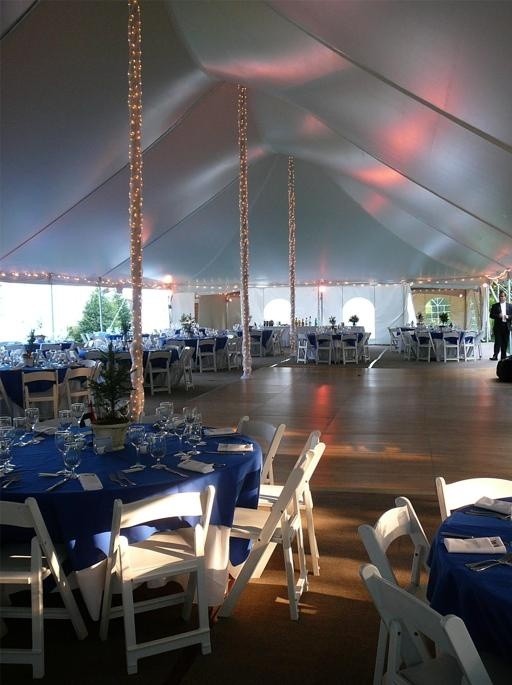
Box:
[490,290,512,360]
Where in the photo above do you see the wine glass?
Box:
[0,325,233,368]
[0,402,205,477]
[314,323,462,334]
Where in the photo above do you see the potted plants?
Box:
[87,344,133,455]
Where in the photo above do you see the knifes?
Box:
[46,477,68,491]
[206,450,245,454]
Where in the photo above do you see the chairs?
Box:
[362,560,491,685]
[216,414,327,623]
[0,497,90,681]
[99,485,217,674]
[295,330,373,368]
[435,477,511,517]
[145,338,216,397]
[358,496,436,685]
[386,327,481,362]
[226,328,285,371]
[1,365,104,422]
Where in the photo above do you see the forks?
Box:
[108,471,137,487]
